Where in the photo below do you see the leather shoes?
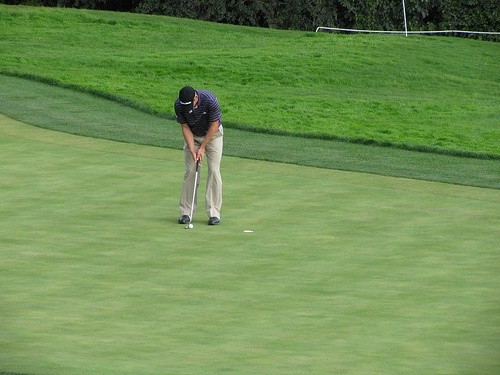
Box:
[179,215,190,224]
[208,217,220,225]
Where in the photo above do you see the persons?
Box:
[173,86,224,225]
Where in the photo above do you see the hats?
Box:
[179,86,195,113]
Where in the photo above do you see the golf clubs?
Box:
[185,158,200,229]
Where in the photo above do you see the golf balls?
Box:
[188,224,194,228]
